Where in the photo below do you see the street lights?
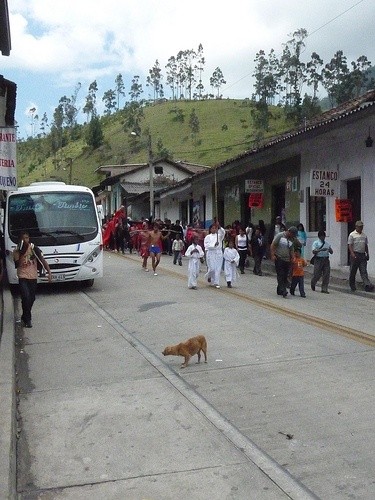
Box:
[130,131,154,218]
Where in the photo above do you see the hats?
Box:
[356,221,363,226]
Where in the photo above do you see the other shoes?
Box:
[179,263,182,266]
[145,268,148,272]
[301,294,306,298]
[240,270,244,274]
[311,282,315,291]
[322,290,329,294]
[208,276,211,283]
[216,285,220,288]
[22,318,32,328]
[283,291,288,297]
[290,290,294,295]
[253,271,263,275]
[154,273,157,276]
[227,282,231,287]
[351,286,356,290]
[193,286,197,290]
[366,286,375,290]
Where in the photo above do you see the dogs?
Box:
[161,334,208,366]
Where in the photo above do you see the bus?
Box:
[1,182,106,288]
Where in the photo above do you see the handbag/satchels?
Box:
[310,256,314,266]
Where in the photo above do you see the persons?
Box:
[102,216,267,259]
[147,223,170,275]
[185,235,204,289]
[0,236,4,281]
[13,231,52,328]
[235,228,251,274]
[347,221,375,292]
[311,231,333,294]
[248,229,267,276]
[139,222,150,272]
[201,221,225,288]
[272,216,286,239]
[223,239,240,288]
[270,223,307,298]
[172,234,185,266]
